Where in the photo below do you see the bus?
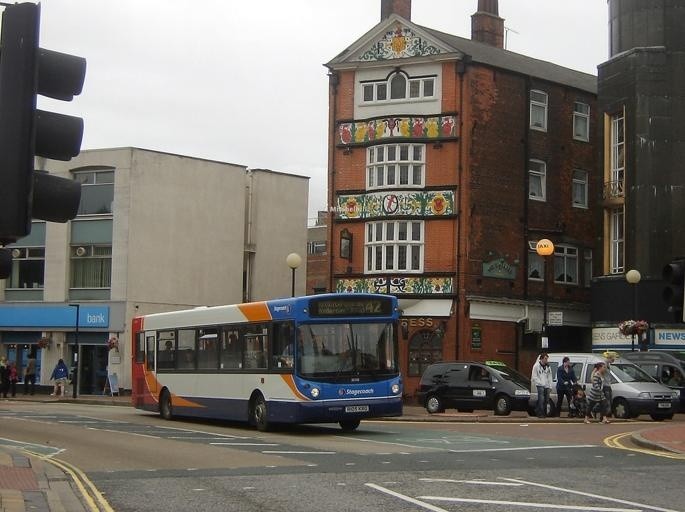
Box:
[130,292,405,434]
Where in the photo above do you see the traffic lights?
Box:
[0,0,87,281]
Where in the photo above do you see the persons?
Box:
[283,326,314,357]
[477,368,489,381]
[49,359,69,398]
[0,354,17,399]
[532,353,615,424]
[23,353,37,396]
[163,341,174,359]
[668,370,681,386]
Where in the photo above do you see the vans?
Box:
[531,352,682,422]
[619,351,685,414]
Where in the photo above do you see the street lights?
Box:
[625,268,642,322]
[286,252,302,299]
[535,238,554,352]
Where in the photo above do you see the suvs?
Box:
[414,360,531,417]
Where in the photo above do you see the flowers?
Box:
[105,336,120,352]
[618,319,648,337]
